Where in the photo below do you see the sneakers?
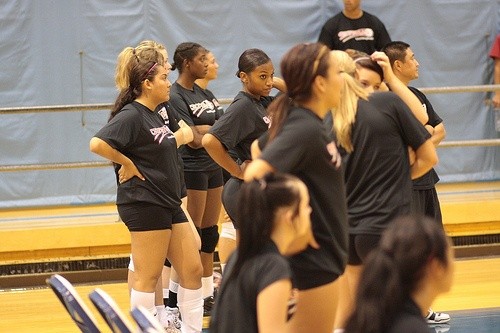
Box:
[424,309,451,324]
[163,320,182,333]
[166,306,182,328]
[204,296,215,316]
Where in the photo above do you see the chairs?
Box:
[46,274,166,333]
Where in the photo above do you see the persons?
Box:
[341,48,430,127]
[341,214,455,333]
[89,38,208,333]
[323,50,442,333]
[193,49,226,120]
[316,0,394,55]
[240,42,349,333]
[210,169,313,333]
[202,48,287,252]
[487,34,500,108]
[163,41,226,317]
[383,40,452,326]
